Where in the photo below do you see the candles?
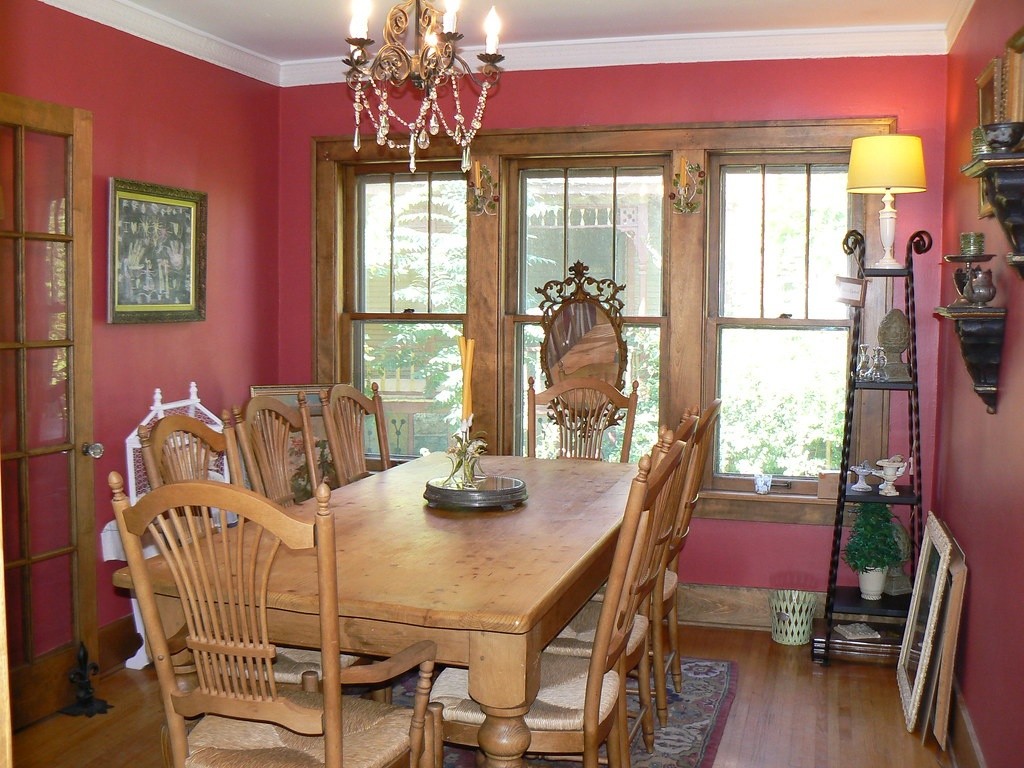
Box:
[680,157,686,187]
[459,335,473,417]
[463,337,474,422]
[960,232,984,256]
[475,160,481,189]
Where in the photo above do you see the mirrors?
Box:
[534,260,628,440]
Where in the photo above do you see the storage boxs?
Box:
[818,469,851,499]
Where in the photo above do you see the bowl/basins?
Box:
[978,122,1024,154]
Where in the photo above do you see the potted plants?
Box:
[841,503,902,601]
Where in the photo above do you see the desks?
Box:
[115,451,640,768]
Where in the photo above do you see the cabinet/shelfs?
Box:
[811,231,934,667]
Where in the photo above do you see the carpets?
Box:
[392,655,740,768]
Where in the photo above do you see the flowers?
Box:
[442,414,487,486]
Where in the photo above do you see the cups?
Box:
[754,474,772,495]
[960,232,984,255]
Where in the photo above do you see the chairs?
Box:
[232,390,319,509]
[109,470,443,767]
[319,382,391,488]
[138,409,373,703]
[429,398,722,766]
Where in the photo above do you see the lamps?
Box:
[342,0,506,172]
[846,134,927,269]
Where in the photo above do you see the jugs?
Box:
[856,343,890,382]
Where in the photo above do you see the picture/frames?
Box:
[897,511,968,753]
[108,176,208,325]
[1001,27,1024,154]
[975,56,994,220]
[834,276,867,308]
[250,385,340,503]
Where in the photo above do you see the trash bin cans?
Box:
[767,589,816,647]
